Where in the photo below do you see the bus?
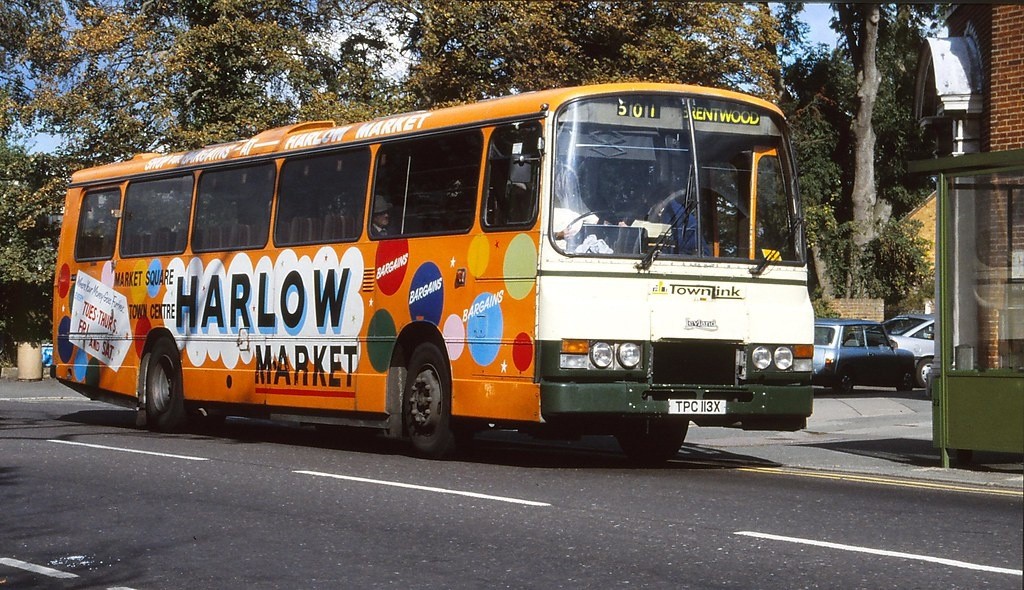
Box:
[48,83,815,459]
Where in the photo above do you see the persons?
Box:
[370,195,395,236]
[554,169,628,240]
[652,183,711,257]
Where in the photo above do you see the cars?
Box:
[815,318,916,398]
[860,314,934,388]
